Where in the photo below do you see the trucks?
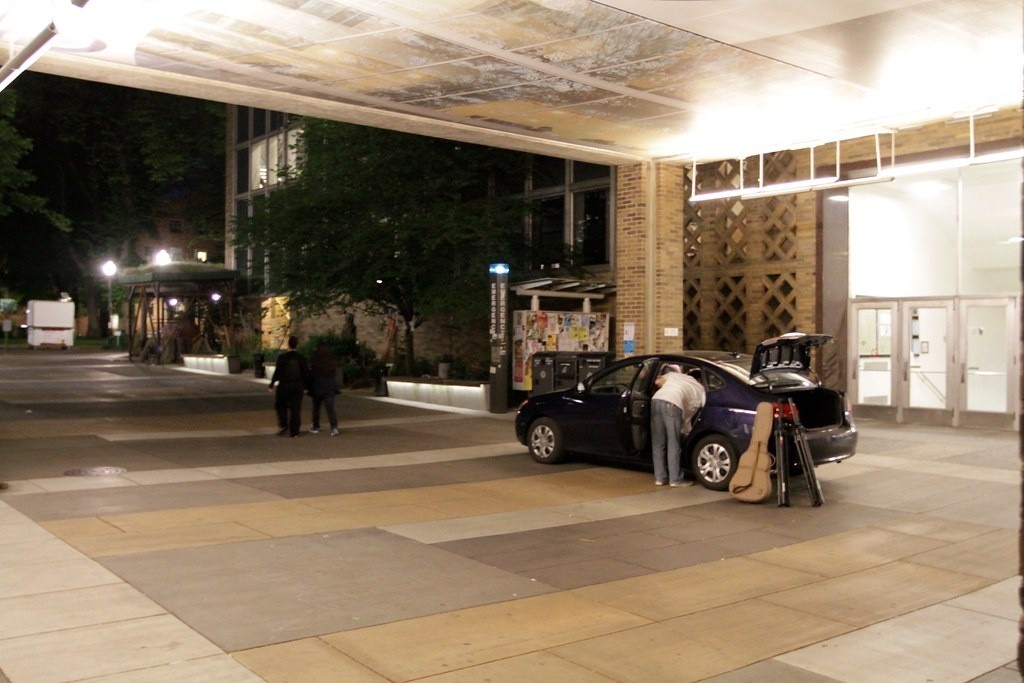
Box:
[27,299,76,352]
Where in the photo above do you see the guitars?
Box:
[729,402,774,503]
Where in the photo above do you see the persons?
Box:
[307,342,339,435]
[651,369,707,487]
[267,336,308,437]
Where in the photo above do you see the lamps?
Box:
[968,116,1024,167]
[687,131,898,201]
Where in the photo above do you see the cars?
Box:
[514,332,858,491]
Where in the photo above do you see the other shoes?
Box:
[278,426,287,434]
[656,480,669,485]
[670,480,693,487]
[331,430,339,436]
[308,428,318,433]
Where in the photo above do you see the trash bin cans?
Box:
[113,331,124,351]
[253,353,265,377]
[436,357,452,379]
[532,349,614,399]
